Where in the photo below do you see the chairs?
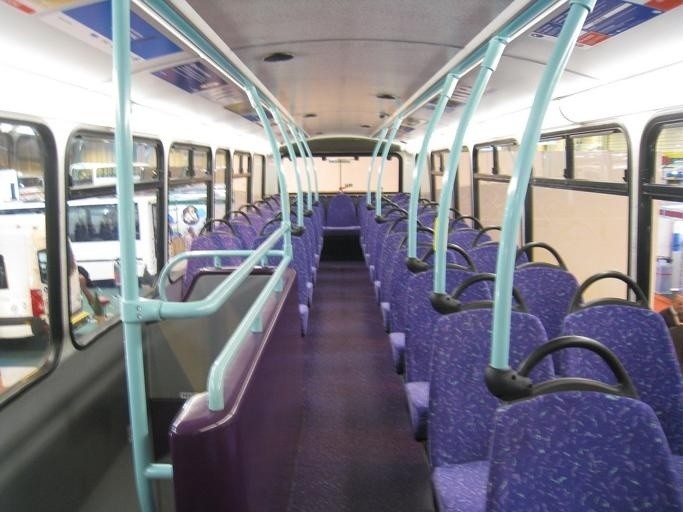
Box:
[324,192,357,234]
[357,188,491,304]
[380,225,586,440]
[425,268,683,507]
[185,192,321,337]
